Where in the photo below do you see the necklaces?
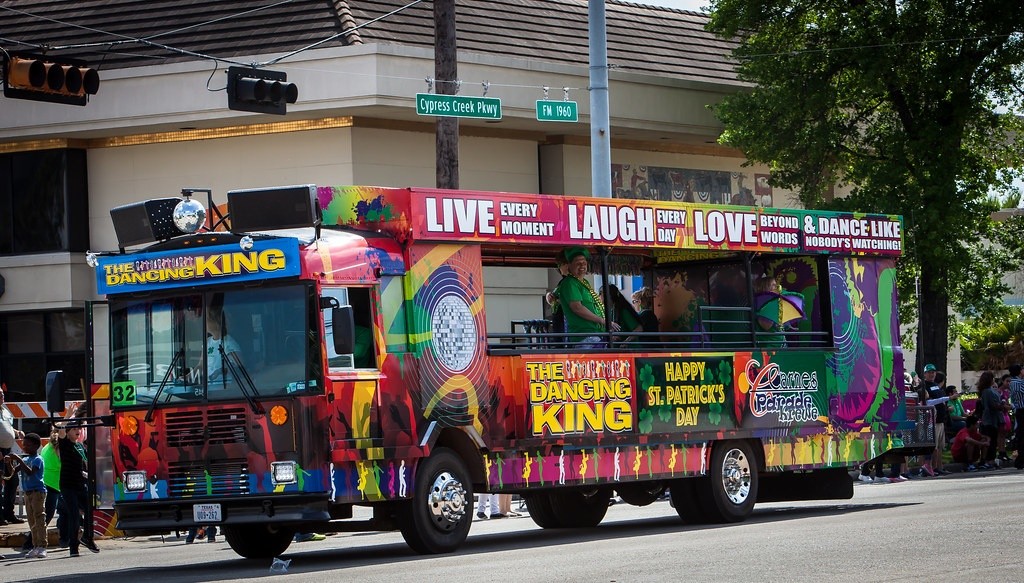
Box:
[570,273,605,319]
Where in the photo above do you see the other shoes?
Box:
[186,533,195,544]
[79,537,100,553]
[903,467,952,478]
[967,454,1013,472]
[38,548,47,557]
[69,546,79,557]
[858,474,873,483]
[873,476,889,482]
[890,474,908,482]
[11,518,25,524]
[0,520,9,525]
[309,532,326,540]
[25,547,39,557]
[208,534,215,542]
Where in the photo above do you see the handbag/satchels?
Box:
[975,399,985,419]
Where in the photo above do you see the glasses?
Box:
[632,299,637,304]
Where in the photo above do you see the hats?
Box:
[923,364,936,373]
[559,247,591,264]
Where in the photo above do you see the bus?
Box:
[46,184,905,560]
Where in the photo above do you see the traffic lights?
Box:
[227,67,298,116]
[4,52,100,106]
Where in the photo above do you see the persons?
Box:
[753,276,787,348]
[858,364,1024,483]
[4,433,49,558]
[180,526,224,544]
[477,493,522,520]
[292,532,326,542]
[632,287,665,352]
[179,303,247,387]
[299,301,373,380]
[557,246,621,353]
[22,401,100,557]
[545,248,573,350]
[0,387,26,526]
[598,283,644,348]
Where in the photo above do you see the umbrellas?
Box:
[753,285,808,331]
